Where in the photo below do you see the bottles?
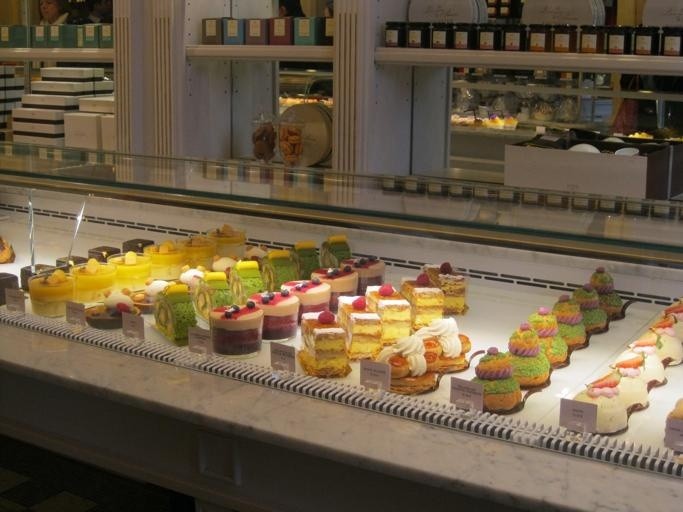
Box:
[251,119,274,165]
[279,122,304,167]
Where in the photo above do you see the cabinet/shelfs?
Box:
[185,1,335,180]
[1,0,115,161]
[375,2,683,240]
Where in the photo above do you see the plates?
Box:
[275,103,332,165]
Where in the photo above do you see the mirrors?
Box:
[230,1,334,18]
[1,2,113,140]
[229,59,333,168]
[411,64,683,204]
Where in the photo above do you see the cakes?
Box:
[210,247,389,355]
[30,219,250,321]
[451,108,518,129]
[0,237,153,318]
[378,312,472,396]
[298,252,473,376]
[154,235,354,345]
[86,244,270,339]
[574,292,683,452]
[474,266,626,412]
